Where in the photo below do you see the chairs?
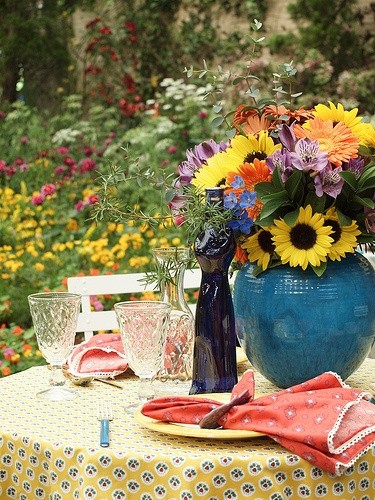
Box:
[66,267,203,344]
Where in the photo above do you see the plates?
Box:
[135,392,268,441]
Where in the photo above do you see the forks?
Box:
[97,413,114,448]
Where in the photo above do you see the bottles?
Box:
[152,248,196,383]
[188,187,239,395]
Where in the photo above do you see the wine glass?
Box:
[114,301,172,414]
[27,291,82,402]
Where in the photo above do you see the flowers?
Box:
[84,19,375,294]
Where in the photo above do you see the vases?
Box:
[187,187,238,395]
[232,249,375,388]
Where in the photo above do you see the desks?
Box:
[0,337,375,500]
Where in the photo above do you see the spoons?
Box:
[63,368,95,385]
